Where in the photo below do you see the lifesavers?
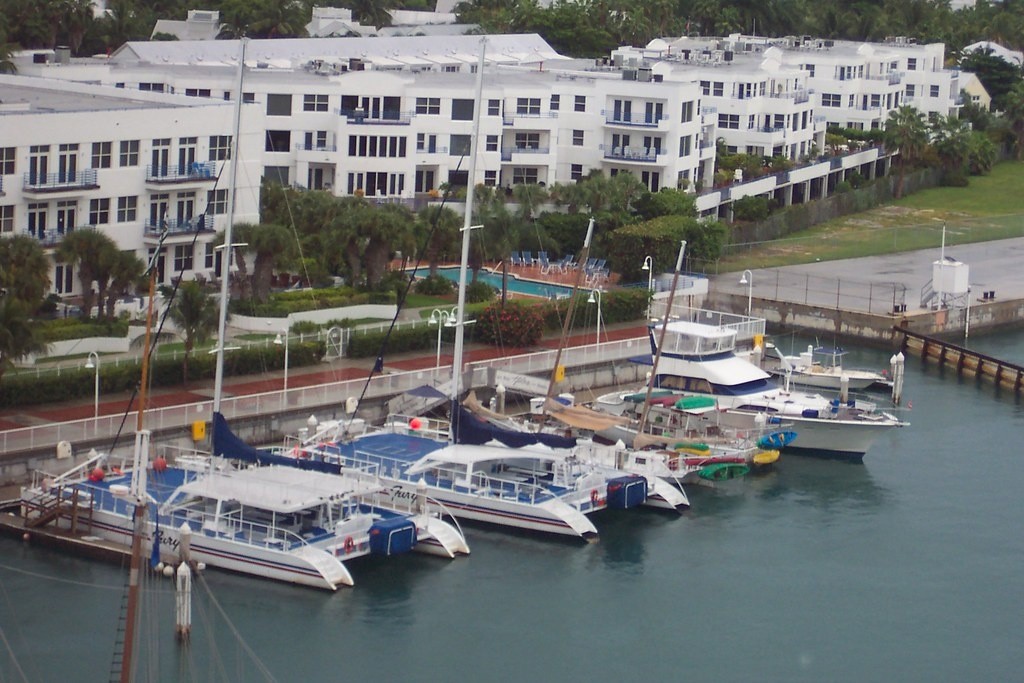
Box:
[344,536,354,554]
[590,489,598,502]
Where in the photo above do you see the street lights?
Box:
[427,309,454,375]
[738,267,753,331]
[586,288,601,359]
[641,253,653,334]
[85,350,100,437]
[273,325,288,408]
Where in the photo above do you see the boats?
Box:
[768,349,886,393]
[588,319,918,464]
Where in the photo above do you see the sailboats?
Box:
[258,29,797,542]
[19,35,473,591]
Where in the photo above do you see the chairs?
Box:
[510,250,549,268]
[195,273,222,285]
[191,162,210,177]
[540,254,611,283]
[614,146,656,160]
